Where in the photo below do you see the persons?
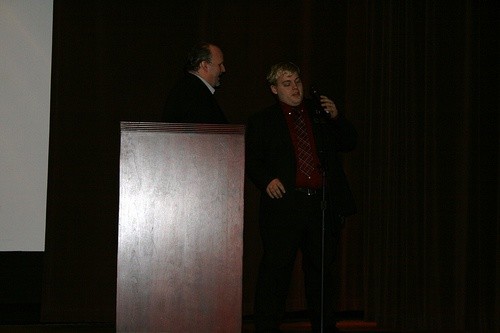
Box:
[246,63,340,331]
[162,44,236,125]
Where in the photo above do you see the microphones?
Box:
[309,86,331,118]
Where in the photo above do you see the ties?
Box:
[293,106,314,180]
